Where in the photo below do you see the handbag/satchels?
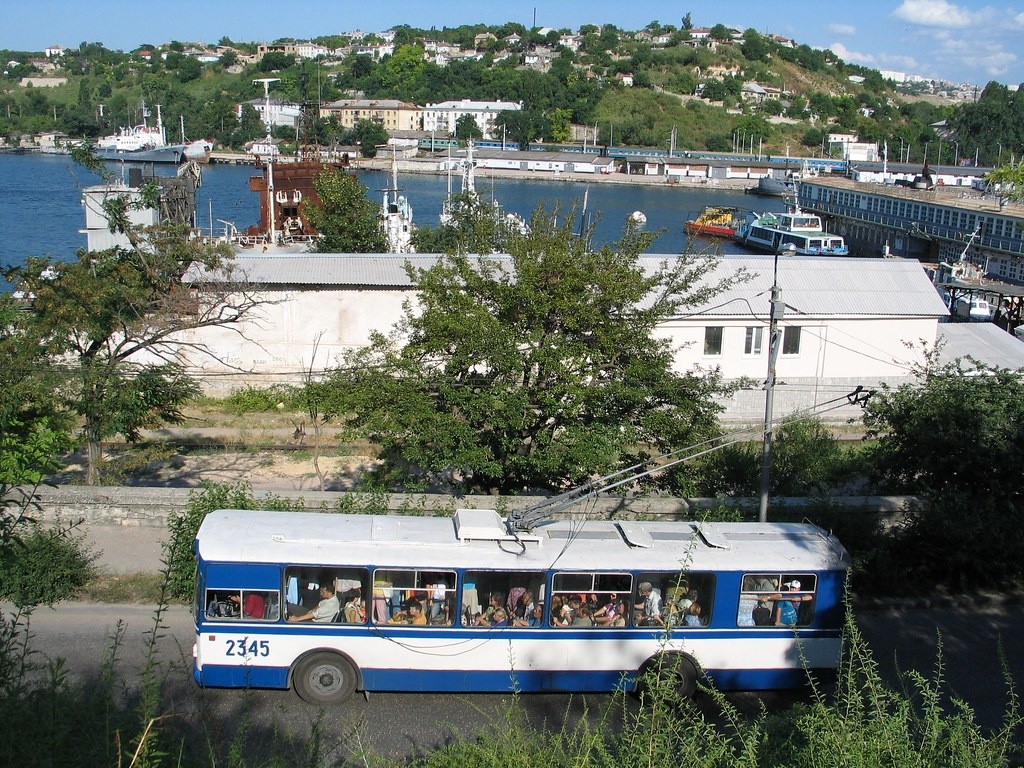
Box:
[752,600,770,626]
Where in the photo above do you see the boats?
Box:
[881,225,1024,343]
[169,114,216,163]
[682,203,761,241]
[217,135,359,254]
[734,173,850,257]
[369,134,421,253]
[92,102,188,164]
[438,133,533,255]
[757,159,821,197]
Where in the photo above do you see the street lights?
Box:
[900,137,903,163]
[610,122,612,147]
[594,120,599,145]
[758,243,796,522]
[954,141,958,167]
[997,142,1001,168]
[743,129,746,153]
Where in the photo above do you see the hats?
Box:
[790,580,801,588]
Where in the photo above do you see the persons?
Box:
[228,575,813,629]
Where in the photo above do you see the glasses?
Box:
[610,593,615,597]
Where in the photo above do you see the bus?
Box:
[188,385,878,707]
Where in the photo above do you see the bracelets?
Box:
[801,597,803,602]
[768,596,770,601]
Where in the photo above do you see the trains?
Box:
[417,138,993,178]
